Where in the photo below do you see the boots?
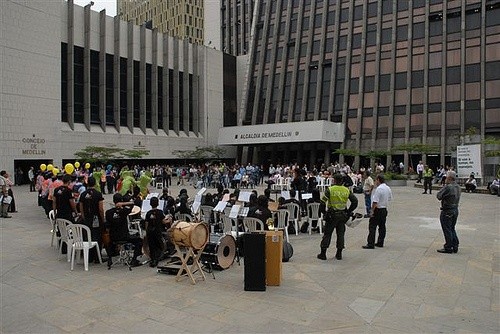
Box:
[422,189,427,194]
[430,189,432,194]
[335,249,342,260]
[317,248,327,260]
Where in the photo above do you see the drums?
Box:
[168,220,209,250]
[201,233,237,271]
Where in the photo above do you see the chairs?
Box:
[271,177,358,193]
[177,203,322,243]
[49,210,103,272]
[106,221,139,271]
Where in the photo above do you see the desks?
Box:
[262,231,283,286]
[242,233,266,291]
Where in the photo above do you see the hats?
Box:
[447,171,456,178]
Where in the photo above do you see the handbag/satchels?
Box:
[0,195,12,205]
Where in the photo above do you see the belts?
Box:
[440,208,457,210]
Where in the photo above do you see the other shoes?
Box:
[149,260,159,267]
[0,214,12,218]
[130,260,139,266]
[437,247,458,254]
[7,210,18,214]
[107,261,113,266]
[362,245,375,249]
[364,215,370,218]
[375,243,383,247]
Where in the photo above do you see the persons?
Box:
[0,160,500,268]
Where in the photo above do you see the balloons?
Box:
[53,168,59,175]
[85,163,91,169]
[65,163,74,174]
[75,162,80,168]
[40,163,46,171]
[46,164,53,171]
